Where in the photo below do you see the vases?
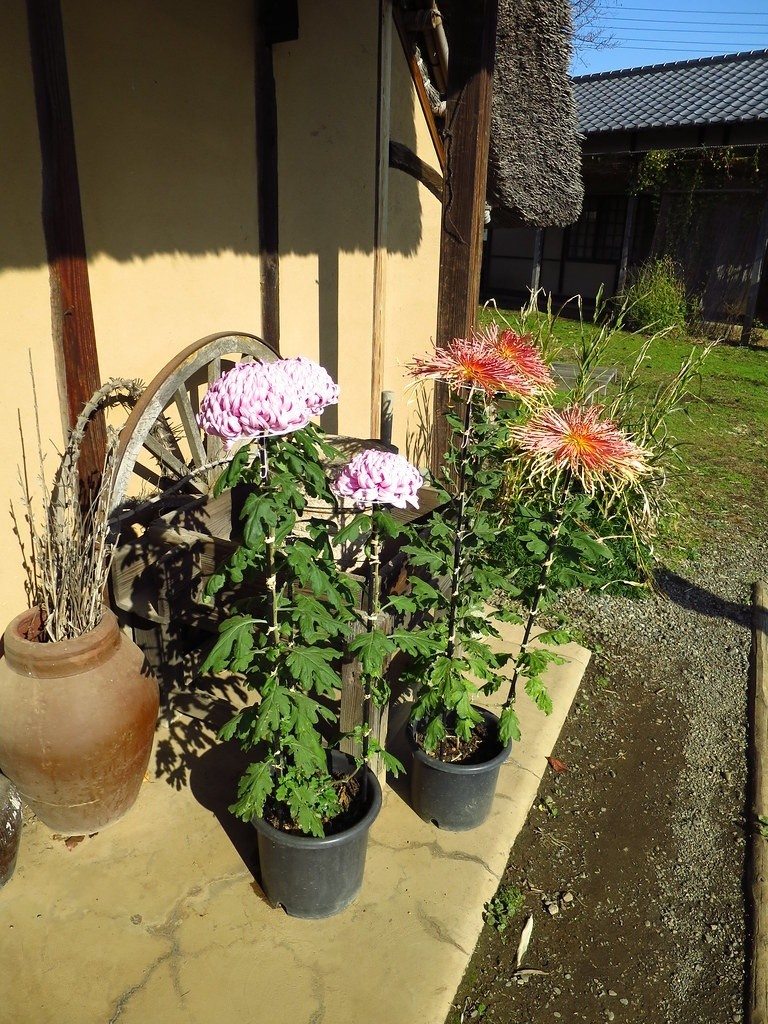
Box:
[405,703,511,830]
[0,601,161,837]
[249,752,384,920]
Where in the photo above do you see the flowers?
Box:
[189,355,422,838]
[384,323,660,756]
